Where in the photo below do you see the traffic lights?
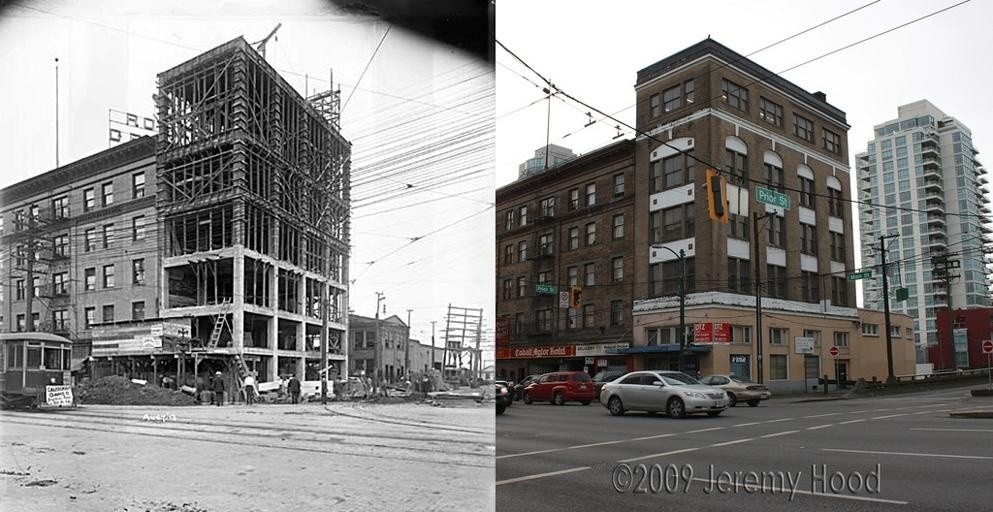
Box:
[571,288,582,309]
[702,170,730,223]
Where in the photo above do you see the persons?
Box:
[211,371,225,406]
[244,373,255,404]
[288,374,301,404]
[321,374,430,405]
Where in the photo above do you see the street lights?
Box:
[652,245,685,373]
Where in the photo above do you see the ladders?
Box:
[207,297,232,350]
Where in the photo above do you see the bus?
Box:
[0,331,77,409]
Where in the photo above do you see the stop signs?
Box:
[830,346,839,356]
[982,340,993,354]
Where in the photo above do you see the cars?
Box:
[495,370,772,419]
